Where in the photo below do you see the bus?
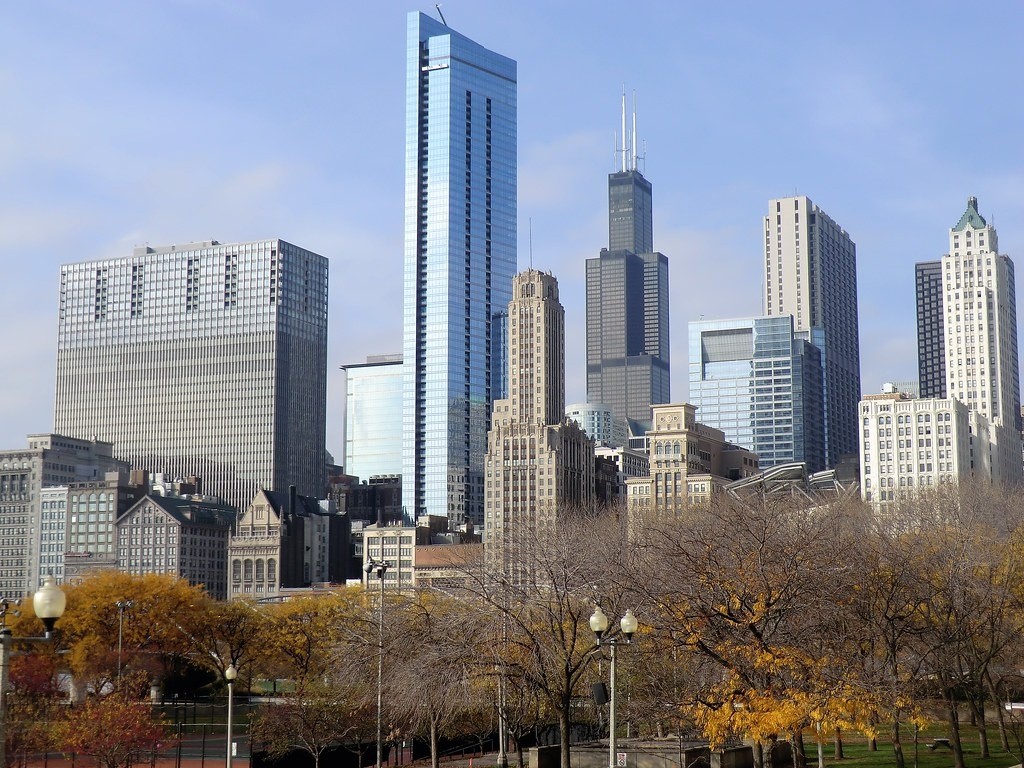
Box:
[5,649,88,709]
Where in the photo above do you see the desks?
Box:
[933,739,950,742]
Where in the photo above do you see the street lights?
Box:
[224,665,239,767]
[363,559,393,768]
[589,607,639,768]
[0,575,69,768]
[115,601,134,696]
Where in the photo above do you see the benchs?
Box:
[926,744,934,753]
[950,744,954,752]
